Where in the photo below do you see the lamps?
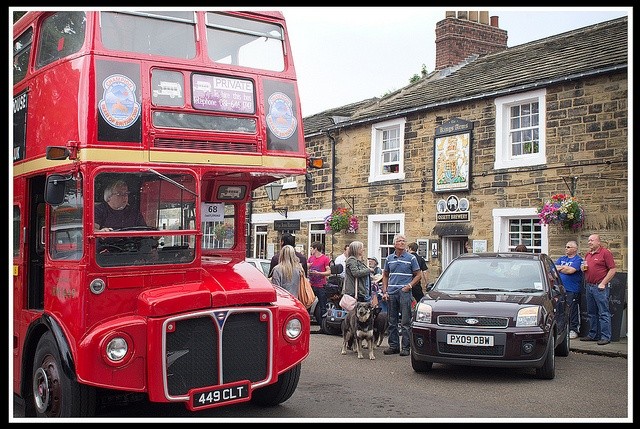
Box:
[264,182,287,216]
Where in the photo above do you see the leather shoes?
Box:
[597,339,608,345]
[581,337,598,341]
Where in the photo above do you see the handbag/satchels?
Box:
[299,271,316,310]
[339,277,358,313]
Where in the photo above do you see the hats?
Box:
[367,257,378,265]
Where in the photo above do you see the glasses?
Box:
[113,191,130,196]
[565,246,575,248]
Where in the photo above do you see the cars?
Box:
[409,253,570,379]
[244,257,320,325]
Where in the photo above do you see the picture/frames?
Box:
[432,132,470,191]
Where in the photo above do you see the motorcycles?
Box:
[322,261,379,334]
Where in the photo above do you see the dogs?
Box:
[372,306,388,347]
[341,298,376,360]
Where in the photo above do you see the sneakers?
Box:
[569,330,578,339]
[400,349,410,356]
[384,348,399,355]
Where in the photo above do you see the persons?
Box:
[270,232,308,279]
[342,240,374,354]
[380,233,423,356]
[514,243,528,252]
[94,179,160,253]
[306,240,331,328]
[554,240,582,340]
[406,242,429,303]
[271,244,305,300]
[367,256,383,288]
[579,234,616,345]
[333,244,349,278]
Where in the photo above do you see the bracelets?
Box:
[407,281,413,291]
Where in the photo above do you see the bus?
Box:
[11,9,311,419]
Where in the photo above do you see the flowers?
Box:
[536,194,583,229]
[324,207,358,233]
